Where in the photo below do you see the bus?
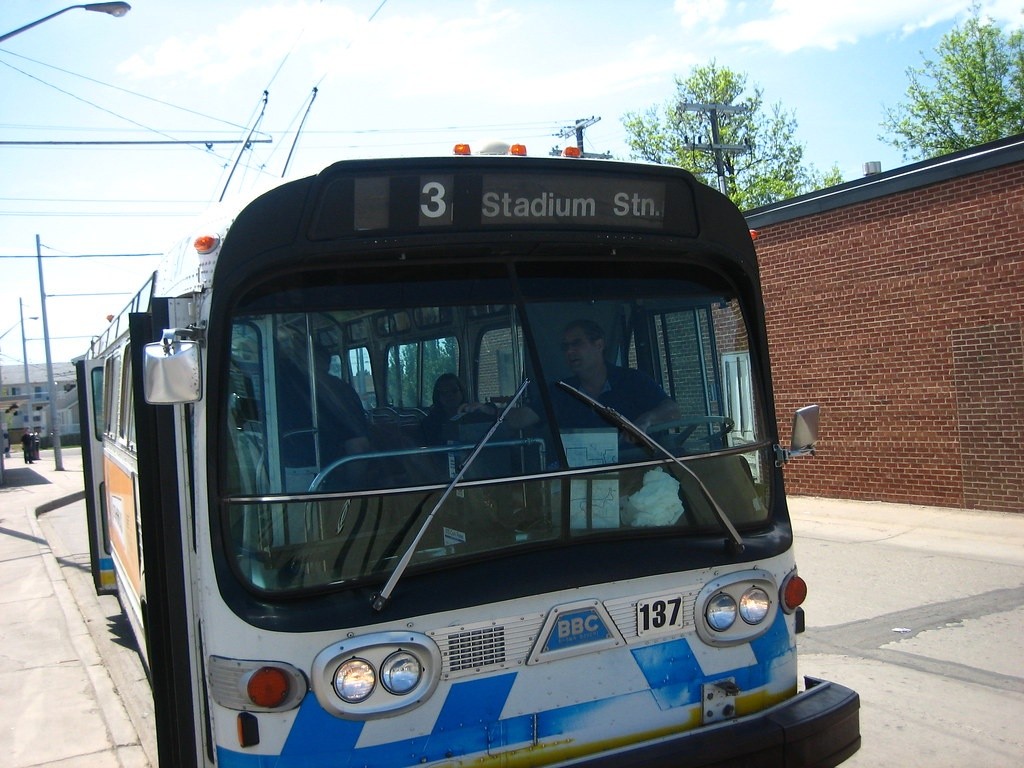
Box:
[69,140,865,767]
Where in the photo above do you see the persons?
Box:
[276,343,370,468]
[21,426,38,463]
[548,319,681,448]
[415,373,498,450]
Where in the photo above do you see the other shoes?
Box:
[25,460,27,464]
[29,462,33,464]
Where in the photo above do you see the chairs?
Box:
[368,403,426,462]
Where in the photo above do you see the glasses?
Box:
[440,387,461,396]
[561,338,591,351]
